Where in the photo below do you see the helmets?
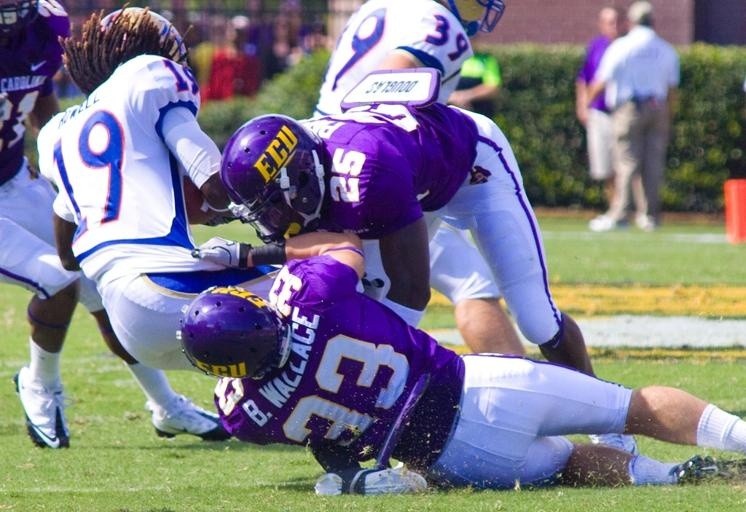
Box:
[218,111,330,247]
[174,283,295,379]
[93,5,190,71]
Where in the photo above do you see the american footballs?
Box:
[184,175,219,225]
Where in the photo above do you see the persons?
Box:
[573,5,629,210]
[449,49,502,121]
[219,99,640,486]
[52,0,335,109]
[583,2,682,232]
[1,1,232,449]
[36,4,429,496]
[312,1,528,357]
[177,233,746,491]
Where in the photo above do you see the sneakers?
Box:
[669,450,746,495]
[589,425,643,459]
[9,366,73,453]
[588,214,663,232]
[142,392,238,443]
